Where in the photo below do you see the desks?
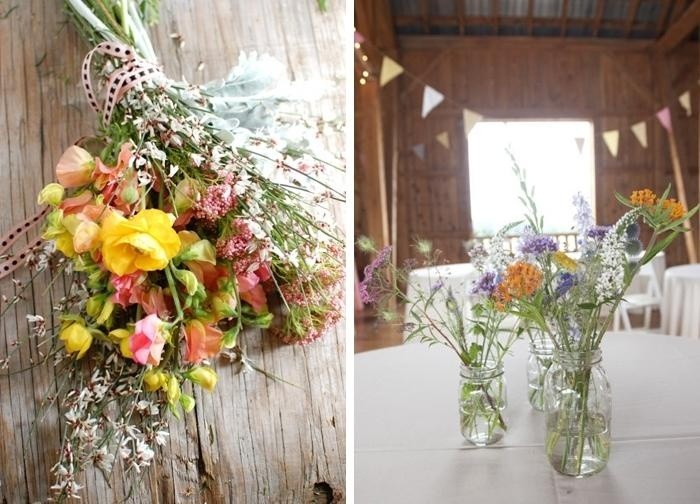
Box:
[354,249,700,499]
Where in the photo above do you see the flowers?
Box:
[357,142,700,477]
[0,0,345,504]
